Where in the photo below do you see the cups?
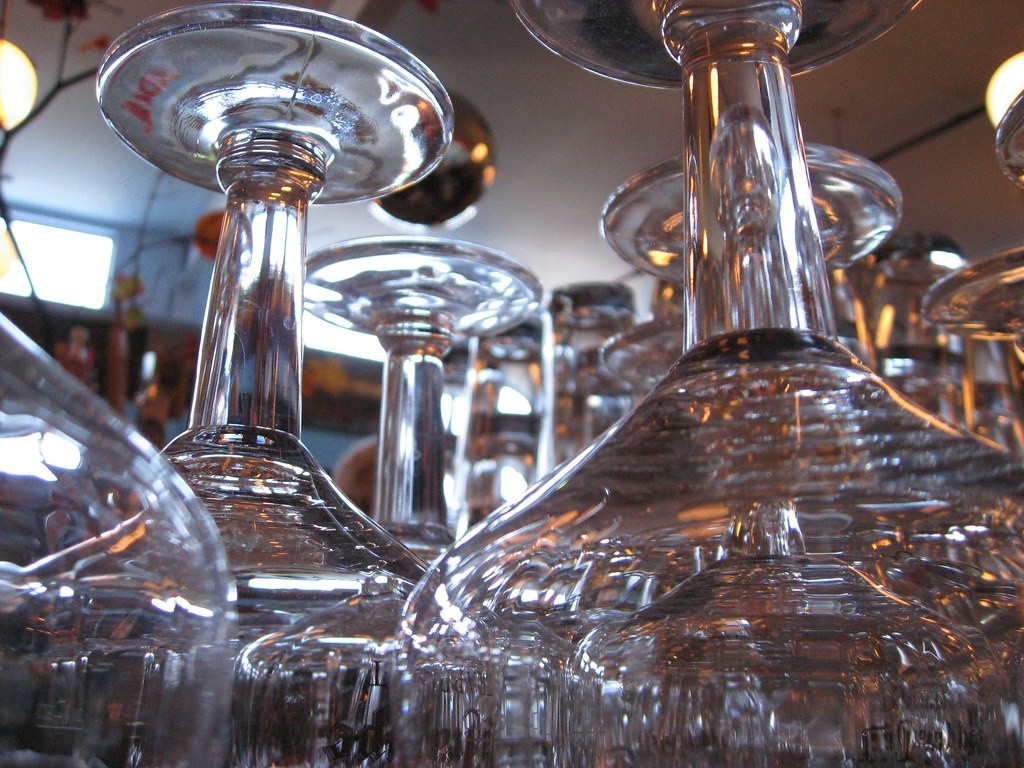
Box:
[544,279,637,464]
[0,311,236,768]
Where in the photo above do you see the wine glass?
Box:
[393,0,1024,768]
[90,1,459,768]
[290,234,546,768]
